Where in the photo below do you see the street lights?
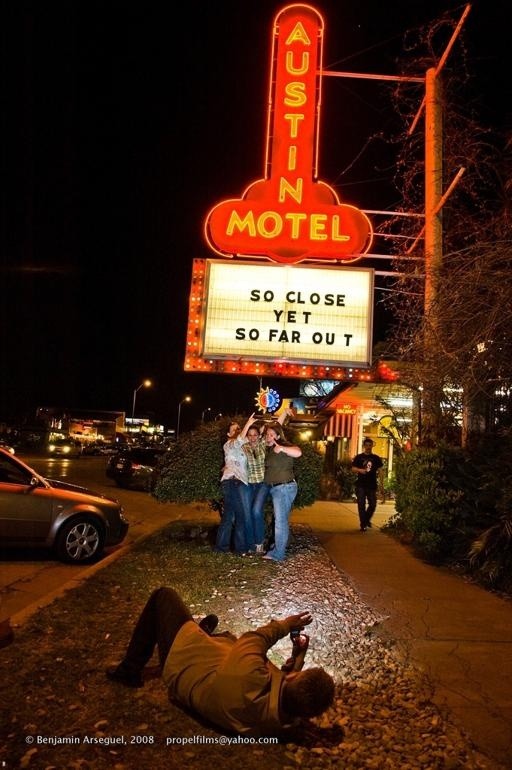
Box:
[131,379,151,432]
[200,407,211,426]
[177,396,191,439]
[215,413,222,421]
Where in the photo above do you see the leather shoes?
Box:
[199,613,218,634]
[105,665,144,688]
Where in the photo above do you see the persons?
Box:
[211,411,269,559]
[260,421,303,563]
[219,408,295,554]
[106,585,334,737]
[351,439,385,532]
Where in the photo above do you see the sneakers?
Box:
[213,544,287,559]
[361,521,371,532]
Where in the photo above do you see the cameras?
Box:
[290,630,300,638]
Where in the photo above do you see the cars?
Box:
[83,434,176,457]
[106,444,170,493]
[0,444,132,568]
[47,438,83,460]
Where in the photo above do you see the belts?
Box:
[270,480,294,488]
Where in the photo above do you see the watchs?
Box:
[280,446,284,455]
